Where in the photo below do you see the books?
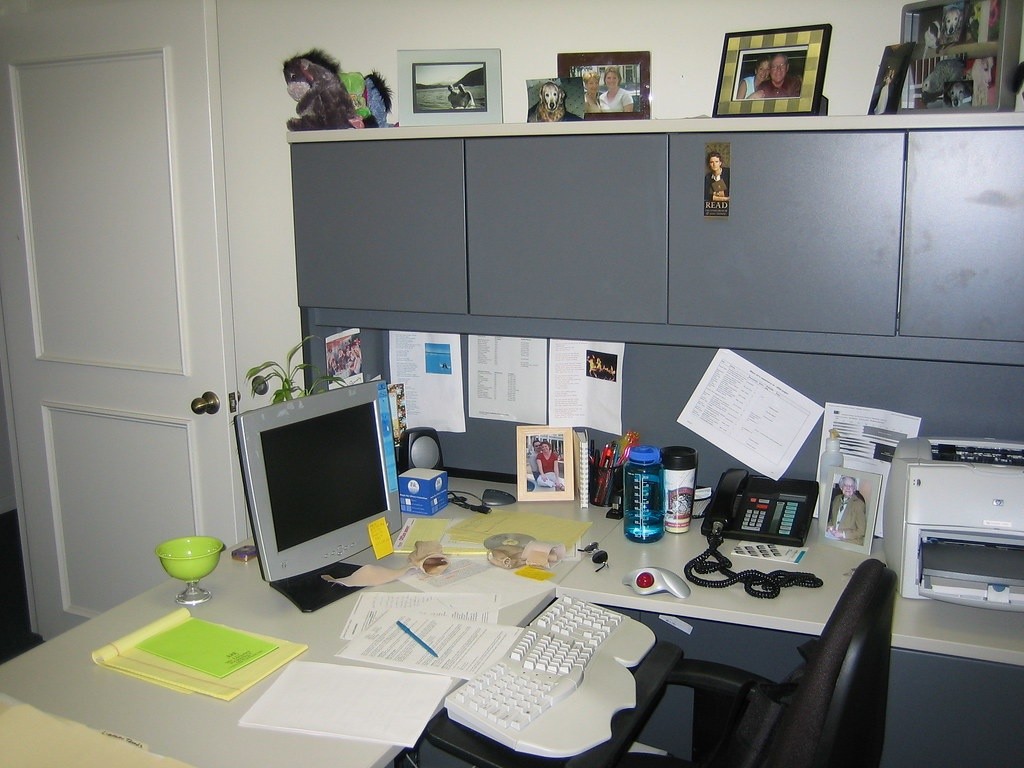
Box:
[92,606,309,700]
[394,517,493,555]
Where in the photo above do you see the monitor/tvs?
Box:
[234,380,402,613]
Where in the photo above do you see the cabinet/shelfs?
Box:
[289,135,675,326]
[652,129,1023,344]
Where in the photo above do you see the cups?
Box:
[660,446,699,534]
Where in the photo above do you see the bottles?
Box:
[622,444,666,544]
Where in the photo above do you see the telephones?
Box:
[701,468,820,548]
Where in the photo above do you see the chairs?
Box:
[395,559,899,768]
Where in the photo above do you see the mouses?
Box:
[622,566,691,598]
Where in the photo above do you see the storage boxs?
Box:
[398,468,449,516]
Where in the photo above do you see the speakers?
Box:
[399,425,444,474]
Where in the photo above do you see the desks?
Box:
[558,507,1024,667]
[0,466,631,767]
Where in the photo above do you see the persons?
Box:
[828,475,867,547]
[327,337,362,383]
[583,72,601,113]
[747,53,801,99]
[737,54,770,99]
[458,84,476,109]
[447,84,460,108]
[537,443,565,491]
[598,67,634,112]
[705,151,730,202]
[530,440,541,481]
[586,354,616,381]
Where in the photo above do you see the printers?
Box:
[882,436,1024,613]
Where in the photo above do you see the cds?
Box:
[484,533,536,550]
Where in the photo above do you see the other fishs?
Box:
[921,58,967,97]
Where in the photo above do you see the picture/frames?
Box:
[820,465,884,557]
[713,24,834,115]
[518,426,575,501]
[555,50,653,120]
[869,40,917,115]
[896,0,1024,116]
[397,48,503,126]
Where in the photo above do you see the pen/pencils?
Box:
[396,620,439,657]
[590,440,622,507]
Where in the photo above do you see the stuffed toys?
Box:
[282,48,399,131]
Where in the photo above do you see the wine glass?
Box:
[156,536,227,605]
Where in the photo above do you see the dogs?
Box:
[924,19,941,54]
[967,57,993,108]
[528,82,567,122]
[942,7,963,43]
[945,82,969,108]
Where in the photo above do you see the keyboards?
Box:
[444,594,655,758]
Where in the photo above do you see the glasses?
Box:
[577,542,608,572]
